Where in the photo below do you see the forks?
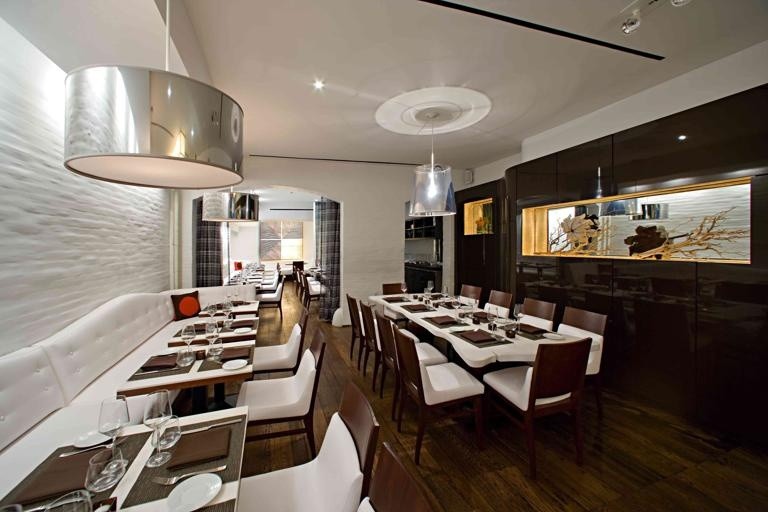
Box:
[55,435,130,460]
[149,464,229,487]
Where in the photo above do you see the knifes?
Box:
[181,417,245,434]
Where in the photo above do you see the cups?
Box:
[506,331,515,339]
[150,416,181,450]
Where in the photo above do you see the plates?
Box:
[69,431,111,447]
[232,327,252,335]
[221,359,248,371]
[543,332,563,341]
[164,472,223,512]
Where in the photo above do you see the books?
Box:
[641,203,668,219]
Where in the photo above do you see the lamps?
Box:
[408,111,457,218]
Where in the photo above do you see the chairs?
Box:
[515,263,763,419]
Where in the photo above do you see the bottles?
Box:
[0,490,94,511]
[84,445,126,493]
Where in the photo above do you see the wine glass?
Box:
[207,286,239,328]
[234,259,260,286]
[176,319,222,367]
[400,280,480,325]
[548,268,717,312]
[513,304,526,336]
[487,306,499,338]
[97,389,174,469]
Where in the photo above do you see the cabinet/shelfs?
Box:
[511,264,763,445]
[516,85,768,210]
[404,216,442,240]
